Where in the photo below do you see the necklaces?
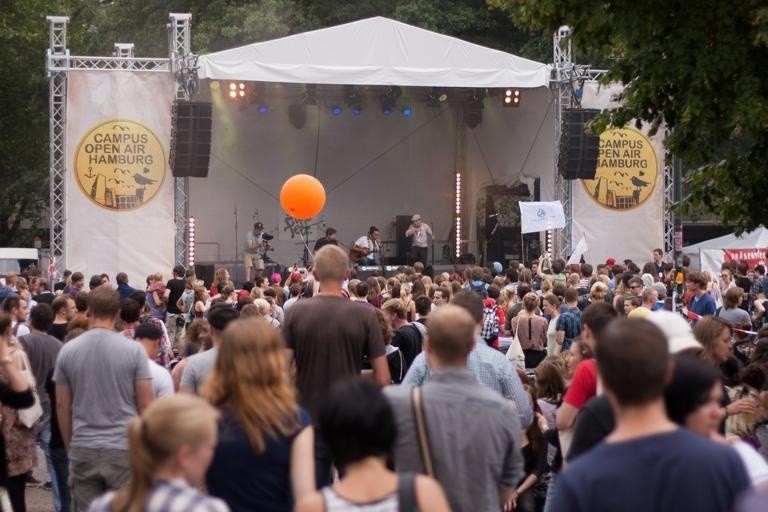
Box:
[415,227,423,238]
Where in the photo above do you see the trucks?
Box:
[0,248,40,295]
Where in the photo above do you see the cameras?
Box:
[261,232,273,240]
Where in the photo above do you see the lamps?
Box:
[227,81,521,118]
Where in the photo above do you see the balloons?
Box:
[278,174,328,220]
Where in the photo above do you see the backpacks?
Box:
[480,301,499,339]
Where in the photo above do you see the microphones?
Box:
[376,237,382,246]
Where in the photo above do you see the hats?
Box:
[411,215,421,221]
[253,223,263,230]
[646,310,704,356]
[292,273,301,281]
[272,273,281,283]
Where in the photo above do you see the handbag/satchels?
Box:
[15,371,43,428]
[176,317,185,327]
[505,336,525,370]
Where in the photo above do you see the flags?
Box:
[566,234,589,264]
[517,200,566,234]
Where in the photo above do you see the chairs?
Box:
[350,241,362,267]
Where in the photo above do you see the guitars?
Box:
[349,245,391,262]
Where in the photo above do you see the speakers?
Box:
[396,215,415,257]
[558,108,601,180]
[357,265,384,281]
[384,265,409,280]
[196,265,214,291]
[168,100,212,178]
[427,265,455,279]
[455,264,476,275]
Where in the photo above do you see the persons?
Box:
[312,227,339,256]
[403,214,436,266]
[351,222,384,267]
[241,221,276,284]
[2,243,768,512]
